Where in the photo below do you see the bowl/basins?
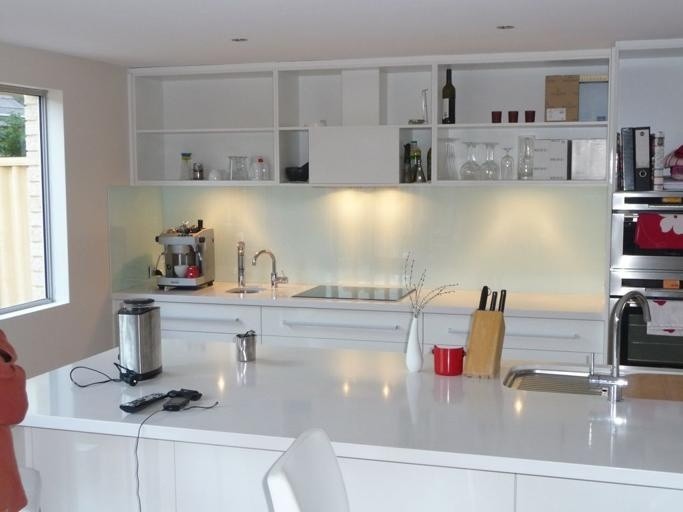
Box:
[285,166,309,182]
[304,120,327,128]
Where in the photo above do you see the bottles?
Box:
[179,152,194,181]
[192,163,204,181]
[402,140,431,183]
[208,169,222,181]
[440,69,456,125]
[249,154,271,183]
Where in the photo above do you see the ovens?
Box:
[608,269,682,370]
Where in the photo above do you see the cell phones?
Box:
[163,396,189,411]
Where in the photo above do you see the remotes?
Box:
[120,393,168,413]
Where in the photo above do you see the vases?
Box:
[405,314,422,372]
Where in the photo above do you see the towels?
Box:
[645,299,682,337]
[631,212,682,251]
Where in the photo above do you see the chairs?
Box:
[261,426,350,512]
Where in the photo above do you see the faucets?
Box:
[251,249,288,288]
[236,241,246,287]
[588,291,651,401]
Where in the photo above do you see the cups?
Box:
[431,372,466,404]
[508,111,518,123]
[431,344,466,376]
[222,154,250,180]
[525,111,535,122]
[492,111,502,123]
[234,333,255,363]
[236,361,257,388]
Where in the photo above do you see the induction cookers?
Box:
[291,284,416,302]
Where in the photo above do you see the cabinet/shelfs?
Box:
[422,307,606,369]
[434,47,613,186]
[261,298,422,351]
[276,54,434,187]
[125,61,276,187]
[109,292,260,349]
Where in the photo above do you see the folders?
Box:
[634,127,651,190]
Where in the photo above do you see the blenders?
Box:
[116,296,164,382]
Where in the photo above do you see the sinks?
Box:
[621,373,683,402]
[226,286,266,294]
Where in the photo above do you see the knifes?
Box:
[490,292,498,311]
[498,289,507,312]
[478,286,489,310]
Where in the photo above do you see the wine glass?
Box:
[437,133,535,181]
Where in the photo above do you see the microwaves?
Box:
[610,191,683,272]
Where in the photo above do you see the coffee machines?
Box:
[153,220,217,292]
